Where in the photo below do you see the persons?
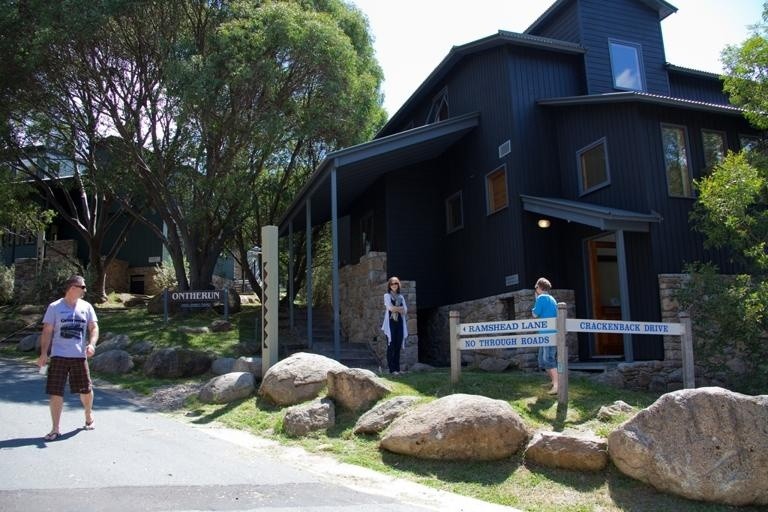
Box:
[37,276,99,442]
[382,277,408,375]
[533,277,560,395]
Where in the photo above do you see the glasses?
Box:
[71,285,86,289]
[390,282,398,285]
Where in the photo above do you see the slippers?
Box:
[85,420,94,430]
[43,432,60,441]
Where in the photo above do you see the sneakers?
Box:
[392,370,404,375]
